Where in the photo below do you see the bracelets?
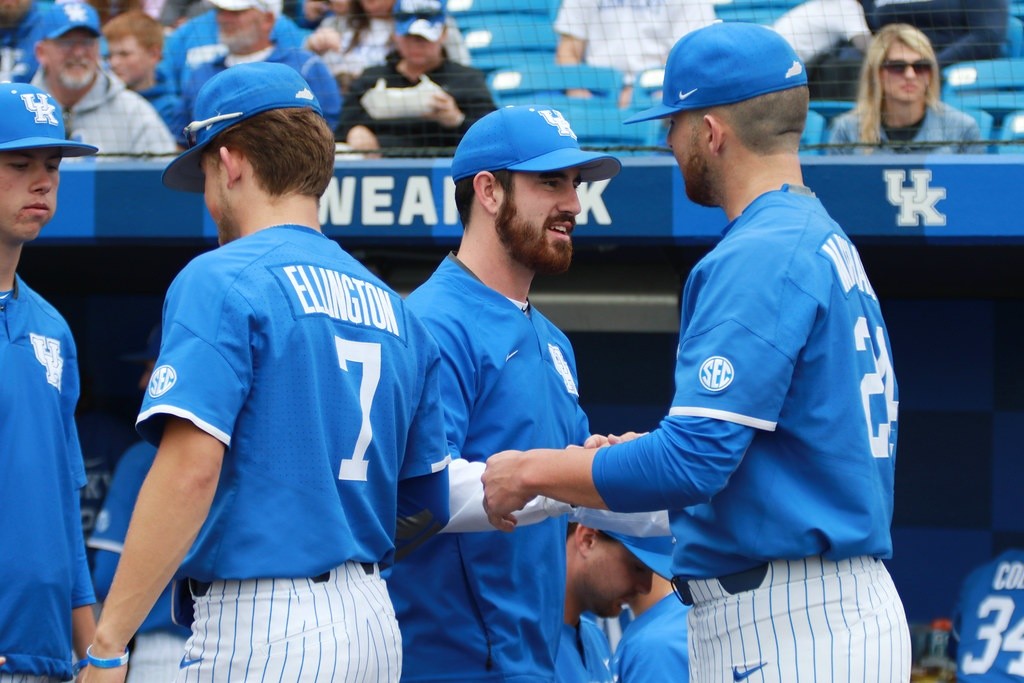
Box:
[71,656,88,677]
[85,641,130,668]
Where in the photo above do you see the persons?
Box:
[0,82,99,683]
[826,21,986,156]
[551,1,717,109]
[554,522,692,682]
[950,548,1024,683]
[769,1,1008,101]
[380,104,651,683]
[0,0,496,158]
[85,321,195,682]
[478,22,912,683]
[68,60,453,683]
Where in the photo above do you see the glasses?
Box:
[881,59,932,74]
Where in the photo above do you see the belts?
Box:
[190,563,374,597]
[674,557,879,604]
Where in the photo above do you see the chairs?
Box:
[447,0,1024,160]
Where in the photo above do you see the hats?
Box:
[0,81,98,157]
[41,0,102,41]
[202,0,283,18]
[121,324,163,361]
[392,0,448,42]
[623,20,807,123]
[569,507,676,583]
[452,105,622,183]
[162,62,323,193]
